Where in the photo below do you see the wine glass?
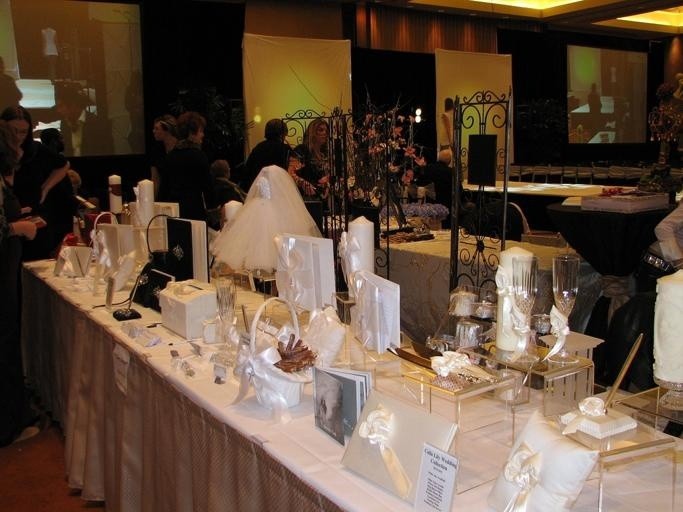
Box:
[512,256,580,366]
[215,274,237,353]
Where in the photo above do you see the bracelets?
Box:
[673,262,683,269]
[298,178,304,187]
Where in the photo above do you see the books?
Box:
[311,366,373,446]
[164,217,211,284]
[96,223,134,267]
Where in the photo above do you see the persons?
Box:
[207,159,244,204]
[438,98,455,167]
[143,115,178,188]
[631,195,682,296]
[286,118,331,235]
[0,104,84,450]
[245,118,292,194]
[35,18,107,157]
[152,111,218,229]
[587,82,603,130]
[422,147,477,226]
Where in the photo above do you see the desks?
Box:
[20,251,683,511]
[377,228,601,345]
[462,181,638,242]
